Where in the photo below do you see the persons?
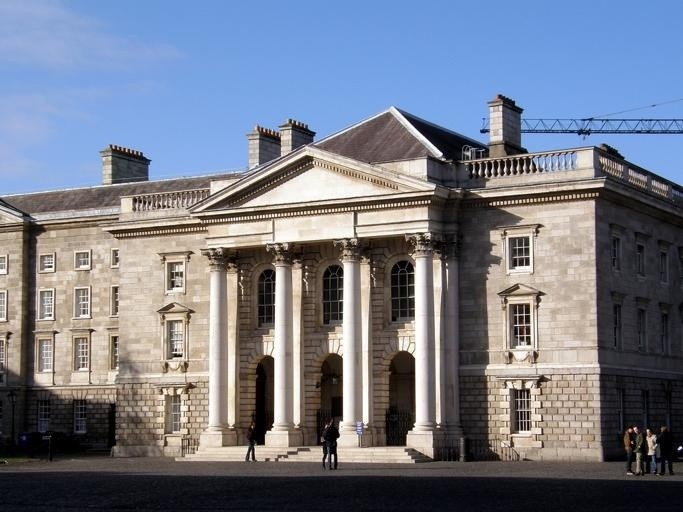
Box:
[646,428,659,474]
[630,425,644,475]
[244,421,258,462]
[319,421,329,470]
[623,425,635,475]
[654,425,675,475]
[323,419,340,470]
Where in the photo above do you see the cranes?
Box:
[479,99,683,141]
